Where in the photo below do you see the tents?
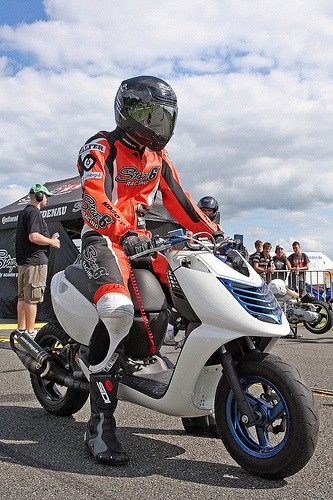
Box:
[1,175,183,240]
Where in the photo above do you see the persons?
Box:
[250,240,310,296]
[196,195,220,223]
[16,184,59,338]
[77,76,246,465]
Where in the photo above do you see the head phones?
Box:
[35,184,43,201]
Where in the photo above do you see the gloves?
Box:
[121,235,155,259]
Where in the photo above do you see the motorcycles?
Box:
[10,230,319,479]
[268,279,333,338]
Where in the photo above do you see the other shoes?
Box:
[181,414,219,437]
[12,329,38,339]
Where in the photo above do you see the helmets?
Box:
[113,76,178,152]
[199,196,219,222]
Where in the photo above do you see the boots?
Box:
[83,369,130,465]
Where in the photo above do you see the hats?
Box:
[28,184,54,197]
[275,244,284,249]
